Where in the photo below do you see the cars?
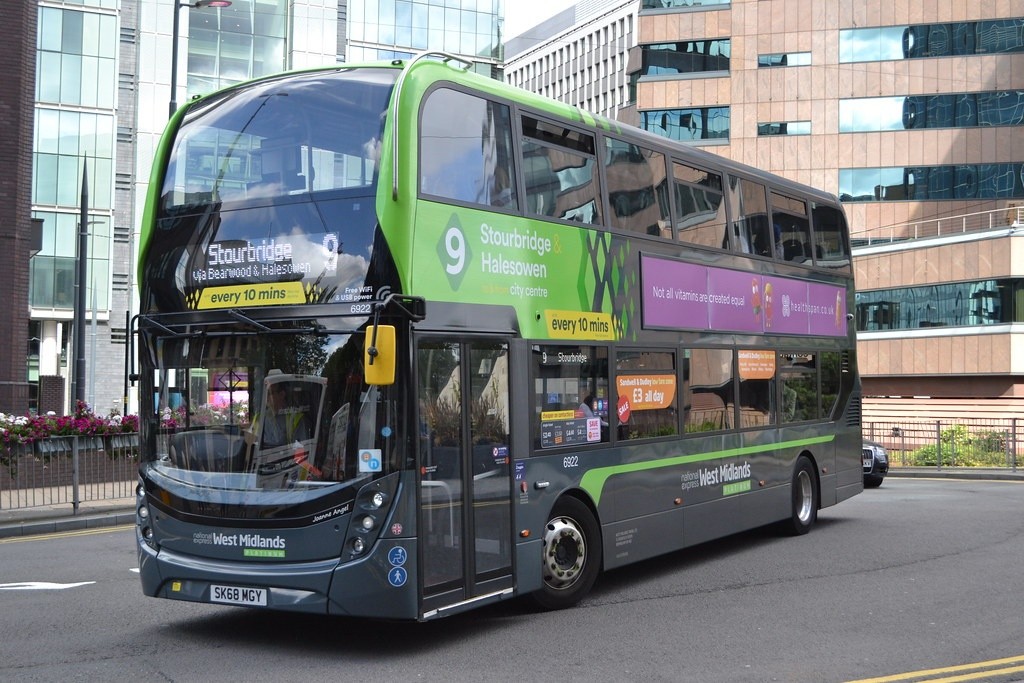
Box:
[861,435,889,488]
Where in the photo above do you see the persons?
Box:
[577,395,593,418]
[779,379,797,424]
[209,382,306,490]
[420,398,438,469]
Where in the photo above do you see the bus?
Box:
[127,50,864,623]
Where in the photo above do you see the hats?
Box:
[268,369,284,376]
